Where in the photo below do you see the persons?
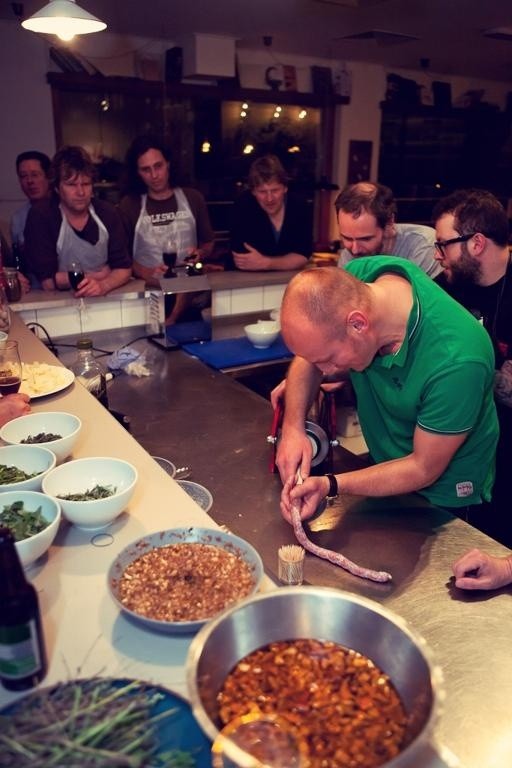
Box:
[24,144,134,299]
[116,137,217,282]
[269,179,449,413]
[268,252,500,529]
[10,149,59,289]
[431,185,512,418]
[221,155,315,273]
[450,547,512,592]
[0,231,33,299]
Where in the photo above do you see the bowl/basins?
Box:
[0,444,56,492]
[0,411,81,464]
[186,584,444,767]
[41,456,138,531]
[0,490,61,572]
[242,322,281,349]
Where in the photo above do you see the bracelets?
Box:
[325,473,338,500]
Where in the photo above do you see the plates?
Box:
[107,526,264,632]
[151,454,176,478]
[1,677,234,767]
[6,363,75,399]
[174,478,214,513]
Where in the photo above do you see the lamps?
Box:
[20,0,108,42]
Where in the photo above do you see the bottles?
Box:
[3,266,21,302]
[69,339,108,409]
[0,527,49,692]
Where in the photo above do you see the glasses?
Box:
[434,234,475,257]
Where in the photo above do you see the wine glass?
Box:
[163,239,178,277]
[65,261,90,310]
[0,339,22,396]
